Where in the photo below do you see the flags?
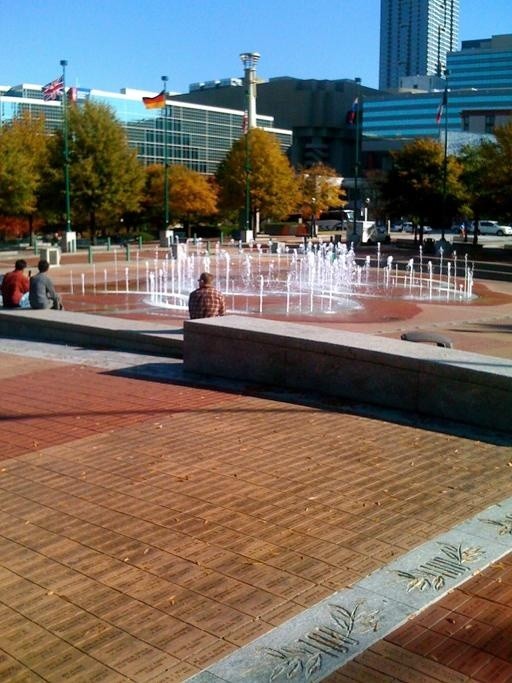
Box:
[141,89,165,109]
[345,97,359,124]
[40,74,65,102]
[241,110,249,134]
[436,91,445,124]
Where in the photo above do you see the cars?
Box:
[377,221,432,233]
[451,220,512,235]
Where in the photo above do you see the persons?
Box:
[2,259,30,308]
[51,232,63,248]
[28,260,64,310]
[188,272,226,320]
[459,225,464,238]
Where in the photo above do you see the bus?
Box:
[304,209,354,230]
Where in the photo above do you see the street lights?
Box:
[240,52,261,234]
